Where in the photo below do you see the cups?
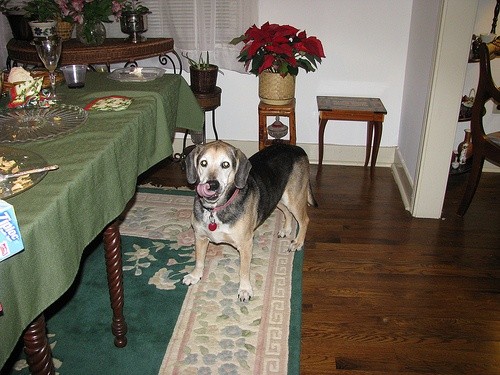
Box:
[59,64,87,89]
[480,33,495,43]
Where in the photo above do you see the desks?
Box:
[258,102,296,150]
[6,34,180,75]
[183,86,222,151]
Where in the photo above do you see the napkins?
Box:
[5,66,33,83]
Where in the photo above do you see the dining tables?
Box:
[0,69,205,375]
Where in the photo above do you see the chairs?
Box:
[455,42,500,218]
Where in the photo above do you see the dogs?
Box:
[182,141,316,302]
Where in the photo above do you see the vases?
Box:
[258,73,295,105]
[56,22,75,41]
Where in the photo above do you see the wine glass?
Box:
[34,37,62,103]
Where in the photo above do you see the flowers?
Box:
[230,21,327,74]
[111,0,127,18]
[55,0,84,21]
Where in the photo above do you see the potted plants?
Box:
[76,0,114,47]
[22,0,60,37]
[181,51,225,93]
[120,0,152,43]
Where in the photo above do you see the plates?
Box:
[106,67,165,83]
[0,146,49,200]
[0,103,88,146]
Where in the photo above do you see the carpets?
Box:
[0,183,303,375]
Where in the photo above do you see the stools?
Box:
[316,96,388,173]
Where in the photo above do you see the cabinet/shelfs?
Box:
[449,35,488,182]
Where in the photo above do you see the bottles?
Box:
[451,129,473,169]
